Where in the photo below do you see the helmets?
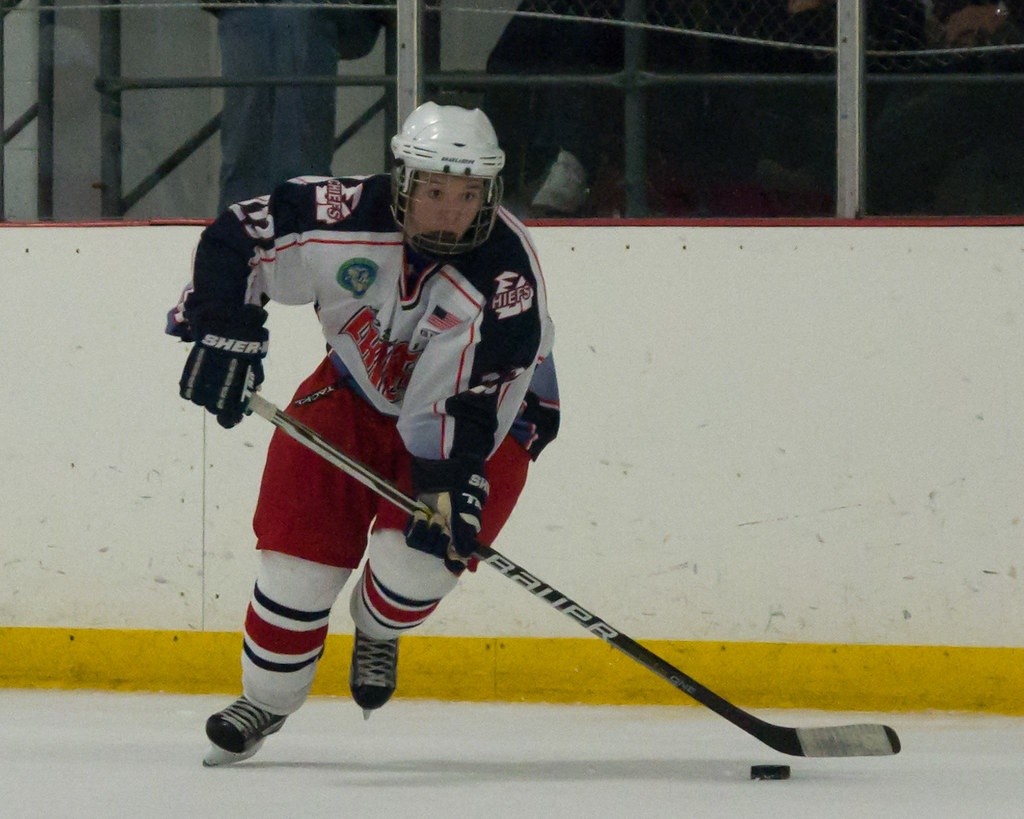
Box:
[390,100,506,254]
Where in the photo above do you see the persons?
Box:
[789,1,1023,212]
[199,1,387,222]
[164,97,565,771]
[511,0,638,222]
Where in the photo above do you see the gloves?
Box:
[179,311,270,427]
[403,459,491,574]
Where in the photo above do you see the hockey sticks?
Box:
[243,388,903,759]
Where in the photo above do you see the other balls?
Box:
[750,764,791,780]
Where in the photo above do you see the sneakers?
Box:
[201,700,283,772]
[347,632,399,717]
